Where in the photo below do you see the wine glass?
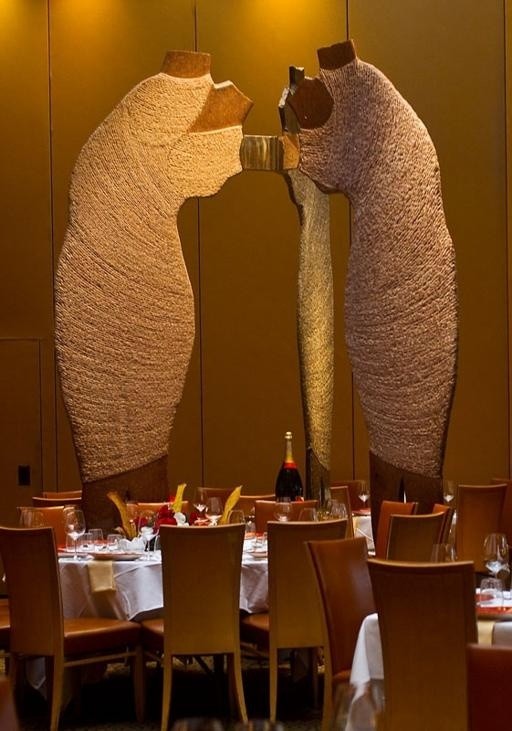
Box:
[63,510,86,562]
[192,487,224,526]
[138,510,160,560]
[358,490,370,511]
[483,534,510,579]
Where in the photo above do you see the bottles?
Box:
[275,431,303,500]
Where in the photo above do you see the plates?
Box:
[90,549,146,561]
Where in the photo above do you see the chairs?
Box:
[303,533,370,729]
[143,524,253,731]
[462,639,512,731]
[363,555,478,730]
[0,477,511,545]
[378,508,445,562]
[1,525,143,731]
[242,516,350,731]
[454,480,508,561]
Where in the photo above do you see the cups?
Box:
[327,498,346,519]
[273,496,294,522]
[479,579,505,608]
[65,528,123,554]
